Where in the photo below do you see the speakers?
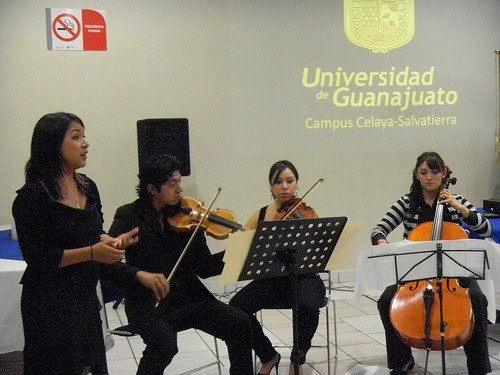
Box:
[137,117,191,180]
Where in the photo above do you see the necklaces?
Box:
[76,199,80,209]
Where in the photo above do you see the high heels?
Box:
[256,352,281,375]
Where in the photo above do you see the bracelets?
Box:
[90,246,93,260]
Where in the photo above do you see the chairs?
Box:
[253,271,339,375]
[101,278,221,375]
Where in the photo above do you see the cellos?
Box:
[387,165,474,374]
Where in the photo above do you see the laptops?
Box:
[482,200,500,215]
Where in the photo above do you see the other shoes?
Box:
[386,355,415,375]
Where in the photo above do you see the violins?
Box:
[273,197,318,221]
[166,196,253,240]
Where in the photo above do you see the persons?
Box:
[230,160,326,375]
[371,151,493,375]
[108,155,252,375]
[12,112,139,375]
